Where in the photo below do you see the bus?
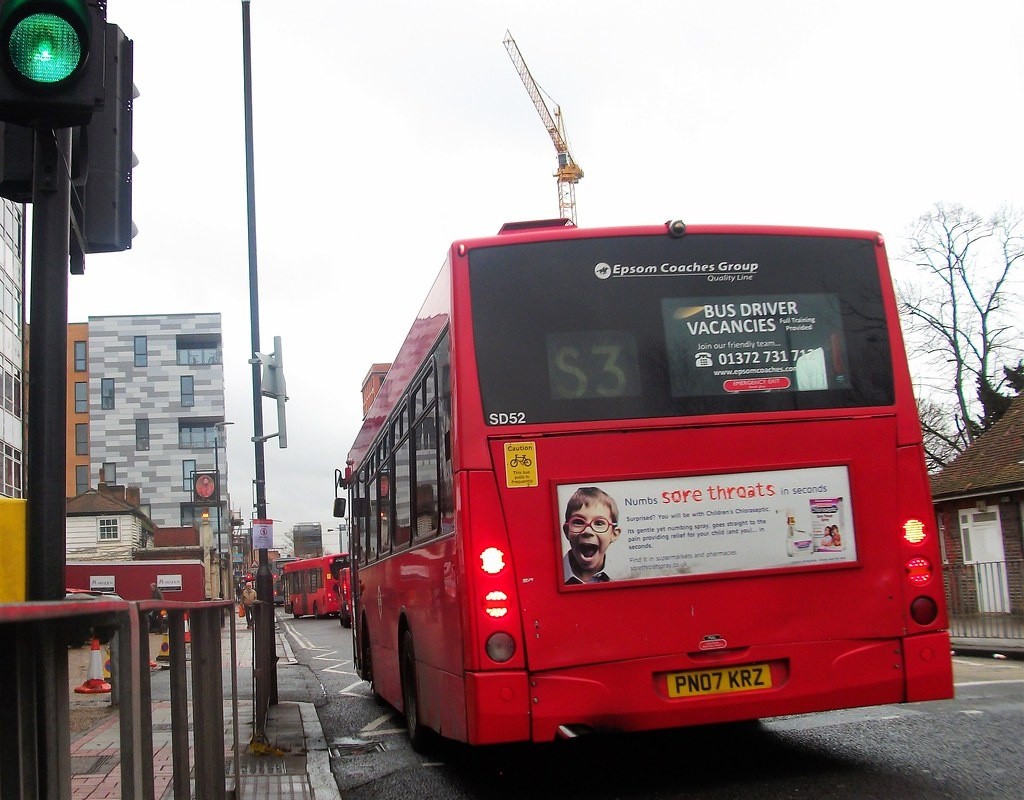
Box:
[279,553,350,619]
[269,558,305,607]
[332,217,956,750]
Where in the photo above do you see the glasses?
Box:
[565,517,618,533]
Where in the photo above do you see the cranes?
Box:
[504,29,585,226]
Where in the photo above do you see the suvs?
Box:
[335,568,351,627]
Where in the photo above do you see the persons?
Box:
[240,582,257,629]
[560,486,626,584]
[147,582,166,632]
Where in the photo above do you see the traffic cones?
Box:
[156,633,170,662]
[75,638,113,697]
[103,643,113,684]
[184,613,192,643]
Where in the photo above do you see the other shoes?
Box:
[247,624,251,628]
[250,623,252,626]
[155,631,161,635]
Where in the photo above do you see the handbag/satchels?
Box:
[238,604,245,617]
